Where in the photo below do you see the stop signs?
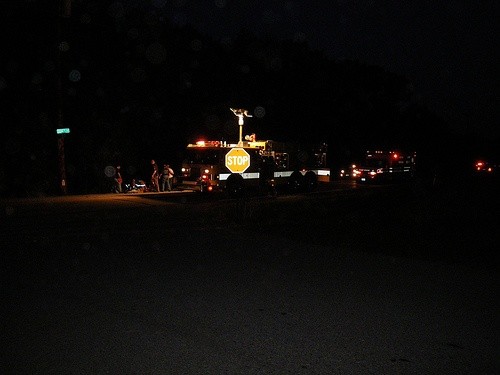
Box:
[225,148,251,174]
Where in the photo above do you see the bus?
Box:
[351,151,416,186]
[181,140,273,186]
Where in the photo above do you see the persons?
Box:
[160,164,174,191]
[151,164,159,192]
[114,165,122,192]
